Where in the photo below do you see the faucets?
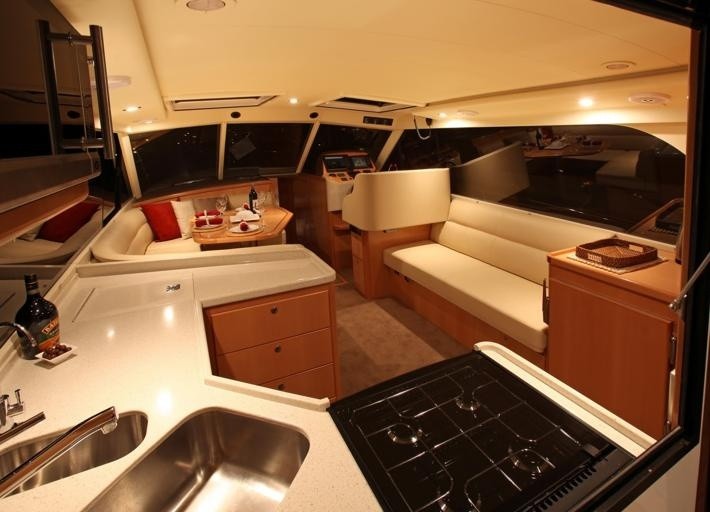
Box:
[1,406,118,499]
[0,412,46,446]
[0,322,37,347]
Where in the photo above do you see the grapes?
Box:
[43,344,71,360]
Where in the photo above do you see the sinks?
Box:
[80,406,311,512]
[0,410,148,498]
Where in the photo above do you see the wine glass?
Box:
[215,191,228,217]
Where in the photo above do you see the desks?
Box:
[188,204,295,248]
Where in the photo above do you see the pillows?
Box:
[140,196,184,242]
[193,194,232,214]
[168,199,197,240]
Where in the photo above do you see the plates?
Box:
[34,343,77,365]
[199,206,263,233]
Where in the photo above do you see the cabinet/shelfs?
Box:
[202,281,341,409]
[545,242,690,441]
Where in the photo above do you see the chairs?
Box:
[340,167,453,231]
[449,138,532,207]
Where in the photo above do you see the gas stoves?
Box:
[328,349,637,512]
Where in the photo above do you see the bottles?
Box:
[248,185,257,213]
[15,273,61,360]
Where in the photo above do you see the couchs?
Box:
[89,202,205,261]
[382,201,614,371]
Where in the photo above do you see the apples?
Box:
[239,222,249,231]
[243,203,249,209]
